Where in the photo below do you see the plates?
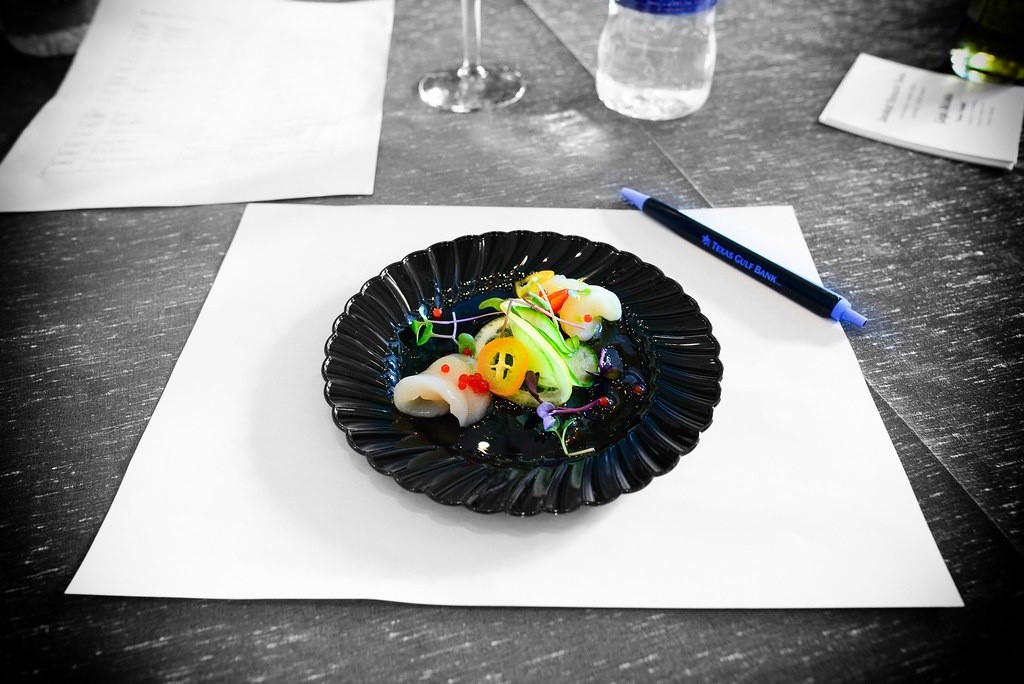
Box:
[322,230,722,517]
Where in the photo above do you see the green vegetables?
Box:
[412,284,596,456]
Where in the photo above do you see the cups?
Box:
[594,0,718,121]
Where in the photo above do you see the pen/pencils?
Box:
[618,187,870,328]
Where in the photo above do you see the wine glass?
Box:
[418,0,526,113]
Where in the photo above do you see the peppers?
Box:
[476,336,528,397]
[515,271,555,297]
